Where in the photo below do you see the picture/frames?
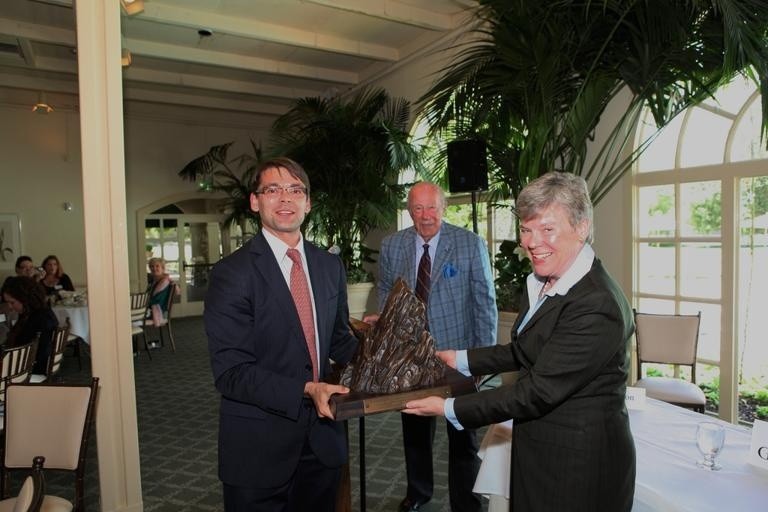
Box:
[0,212,23,274]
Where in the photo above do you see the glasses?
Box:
[253,186,308,195]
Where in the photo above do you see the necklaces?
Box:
[542,274,551,297]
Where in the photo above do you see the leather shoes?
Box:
[398,495,431,512]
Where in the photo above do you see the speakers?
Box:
[445,138,489,195]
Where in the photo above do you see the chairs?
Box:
[129,289,152,361]
[0,377,99,512]
[13,455,46,512]
[29,317,71,386]
[136,283,176,358]
[0,332,40,434]
[632,308,706,415]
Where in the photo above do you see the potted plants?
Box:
[177,84,419,323]
[419,0,768,347]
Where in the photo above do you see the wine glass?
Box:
[694,421,726,472]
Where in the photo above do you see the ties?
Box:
[537,278,553,299]
[285,247,318,383]
[413,243,432,308]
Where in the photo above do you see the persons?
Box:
[360,182,498,512]
[1,276,59,384]
[399,171,636,512]
[204,156,362,512]
[16,256,46,302]
[39,257,75,301]
[133,258,171,358]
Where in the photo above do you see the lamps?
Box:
[122,47,132,66]
[120,0,145,17]
[33,92,54,115]
